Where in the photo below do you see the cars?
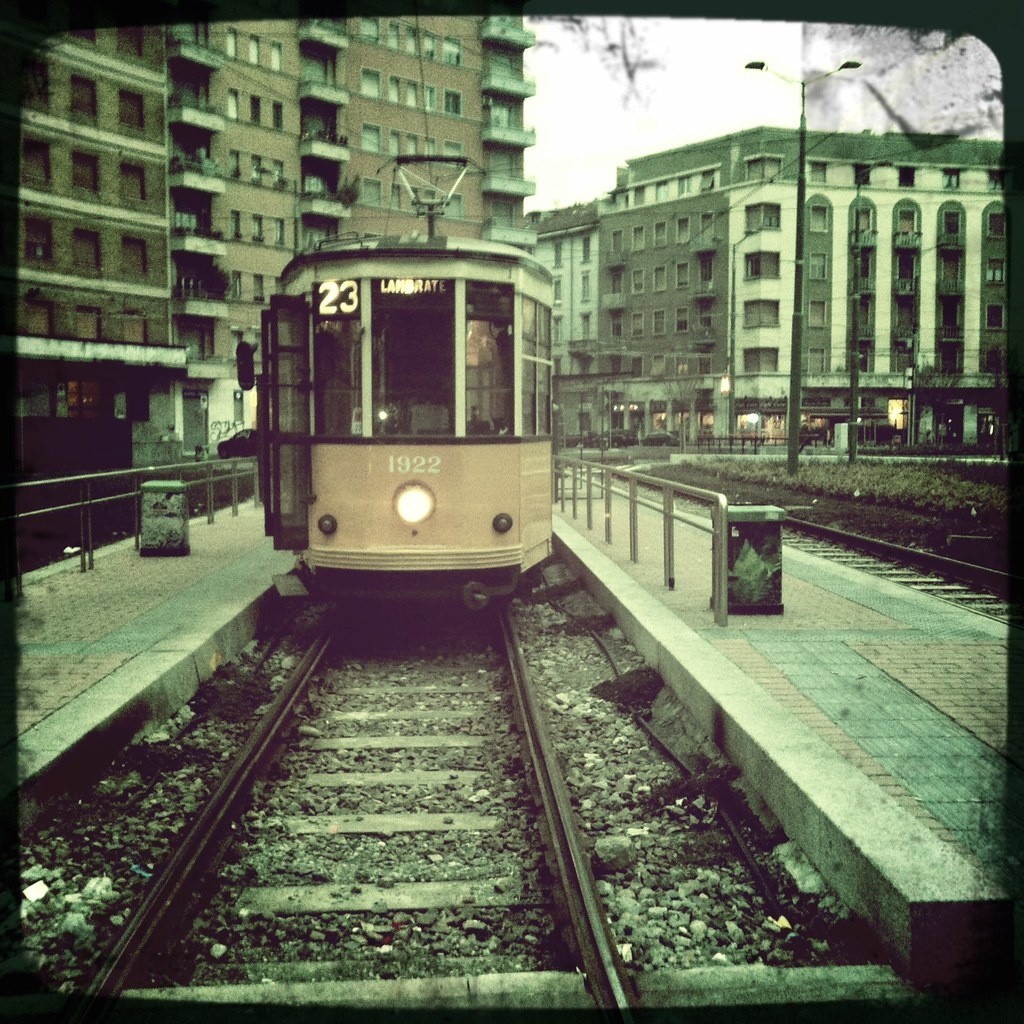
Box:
[216,428,256,460]
[596,428,680,449]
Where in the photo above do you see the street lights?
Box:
[744,60,863,475]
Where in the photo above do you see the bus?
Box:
[254,153,556,600]
